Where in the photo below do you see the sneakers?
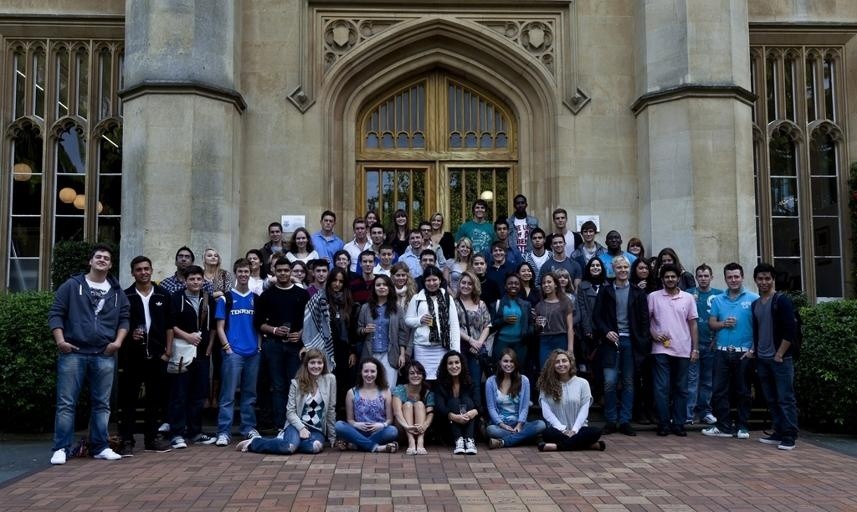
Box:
[52,448,66,462]
[172,438,187,449]
[217,434,229,446]
[241,430,259,440]
[194,434,215,445]
[236,436,257,451]
[93,448,123,460]
[778,440,794,449]
[700,415,717,424]
[758,436,782,444]
[737,429,749,439]
[453,437,465,454]
[701,426,733,437]
[464,438,477,455]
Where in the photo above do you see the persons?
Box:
[335,357,398,453]
[300,192,575,407]
[236,348,337,455]
[752,262,803,450]
[391,360,435,455]
[435,350,481,455]
[575,220,723,437]
[701,262,761,439]
[485,348,546,450]
[117,223,301,454]
[537,348,605,450]
[49,246,131,465]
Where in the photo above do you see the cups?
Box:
[427,317,433,326]
[369,324,375,339]
[727,312,736,325]
[511,310,517,320]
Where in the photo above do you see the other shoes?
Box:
[618,423,633,435]
[417,446,428,455]
[600,422,618,434]
[144,440,172,454]
[121,445,133,457]
[489,438,503,449]
[406,447,417,456]
[538,442,546,452]
[336,441,358,450]
[670,424,687,436]
[158,422,170,432]
[656,424,669,436]
[385,441,399,454]
[596,441,605,451]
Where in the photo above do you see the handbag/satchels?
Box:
[479,344,489,369]
[167,338,197,373]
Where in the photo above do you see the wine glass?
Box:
[282,322,291,342]
[615,340,622,354]
[541,316,547,333]
[138,325,147,344]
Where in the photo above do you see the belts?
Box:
[717,344,750,353]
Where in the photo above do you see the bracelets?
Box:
[382,421,388,428]
